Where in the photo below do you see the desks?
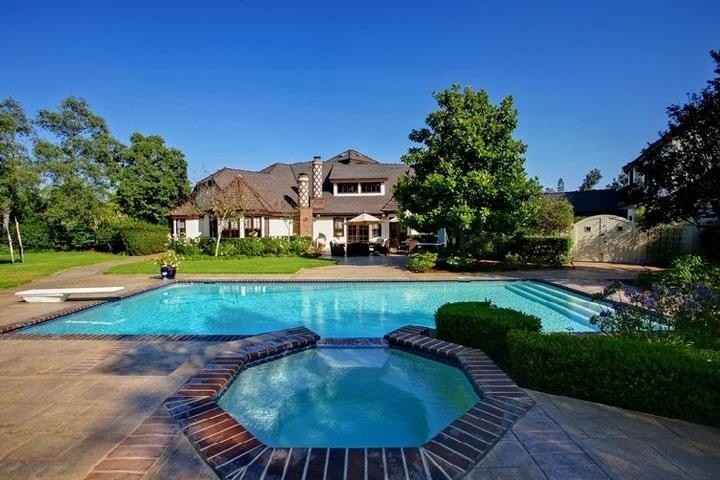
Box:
[404,241,443,252]
[368,243,376,253]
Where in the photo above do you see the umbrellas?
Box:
[345,209,384,244]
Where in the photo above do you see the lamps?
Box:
[381,213,388,221]
[312,214,320,222]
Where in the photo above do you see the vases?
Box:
[161,267,177,278]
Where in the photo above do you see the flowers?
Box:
[152,253,183,269]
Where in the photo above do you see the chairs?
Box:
[389,237,407,255]
[374,239,390,256]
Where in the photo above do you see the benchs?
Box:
[330,240,346,258]
[347,242,370,257]
